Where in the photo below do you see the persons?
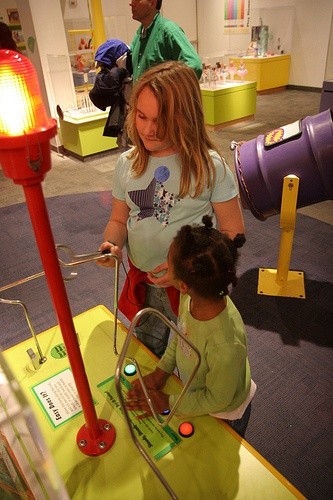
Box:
[97,61,247,377]
[113,0,203,156]
[0,20,19,52]
[124,214,257,441]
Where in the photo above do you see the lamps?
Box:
[0,48,58,187]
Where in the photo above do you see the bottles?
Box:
[124,364,139,384]
[259,27,269,57]
[202,61,227,88]
